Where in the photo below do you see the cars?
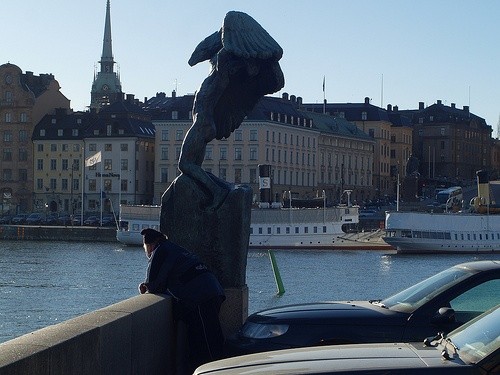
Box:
[0,213,83,227]
[97,217,113,226]
[191,302,500,375]
[84,216,100,226]
[238,260,500,356]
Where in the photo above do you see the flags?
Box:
[85,151,101,167]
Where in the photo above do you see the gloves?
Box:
[139,282,147,294]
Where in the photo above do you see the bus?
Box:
[434,186,463,209]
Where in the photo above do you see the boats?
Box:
[114,202,362,246]
[380,172,500,255]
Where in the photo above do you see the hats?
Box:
[141,228,166,244]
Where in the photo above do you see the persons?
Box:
[139,230,226,369]
[176,49,254,215]
